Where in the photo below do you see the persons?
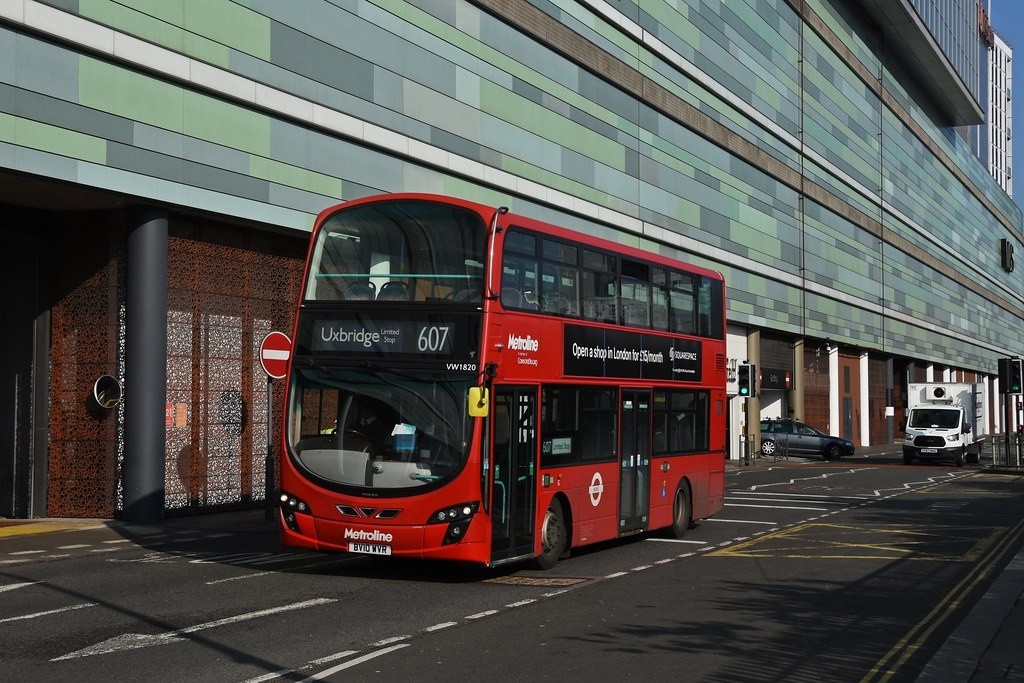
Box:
[348,397,386,440]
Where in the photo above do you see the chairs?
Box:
[342,281,693,335]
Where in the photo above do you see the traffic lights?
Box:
[1018,402,1023,409]
[1010,359,1022,394]
[737,364,752,398]
[1018,425,1023,436]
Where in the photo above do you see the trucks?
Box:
[898,383,986,467]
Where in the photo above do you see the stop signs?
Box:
[786,372,790,387]
[759,371,762,384]
[261,331,291,380]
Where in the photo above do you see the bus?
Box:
[276,192,726,564]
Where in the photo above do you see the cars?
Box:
[760,420,855,461]
